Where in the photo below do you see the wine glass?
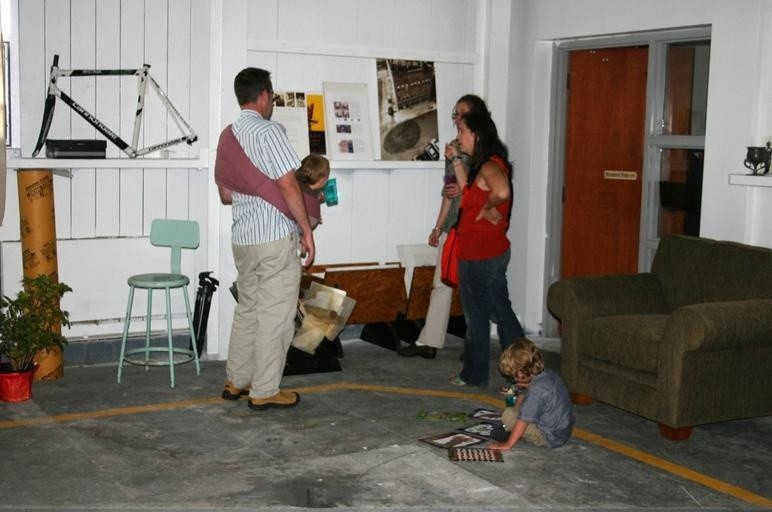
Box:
[745,147,767,176]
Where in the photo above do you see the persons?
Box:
[444,111,526,387]
[484,337,573,451]
[214,68,315,410]
[398,94,491,359]
[229,154,329,301]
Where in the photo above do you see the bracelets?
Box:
[451,156,461,161]
[432,225,443,232]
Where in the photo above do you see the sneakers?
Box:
[222,379,252,400]
[249,391,300,410]
[498,384,521,397]
[488,426,516,443]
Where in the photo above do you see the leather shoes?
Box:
[396,343,439,360]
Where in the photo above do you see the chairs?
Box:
[544,231,771,441]
[116,218,203,388]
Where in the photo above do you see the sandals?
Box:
[448,371,466,388]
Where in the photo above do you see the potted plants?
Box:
[0,272,74,403]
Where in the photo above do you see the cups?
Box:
[443,173,455,183]
[321,179,339,207]
